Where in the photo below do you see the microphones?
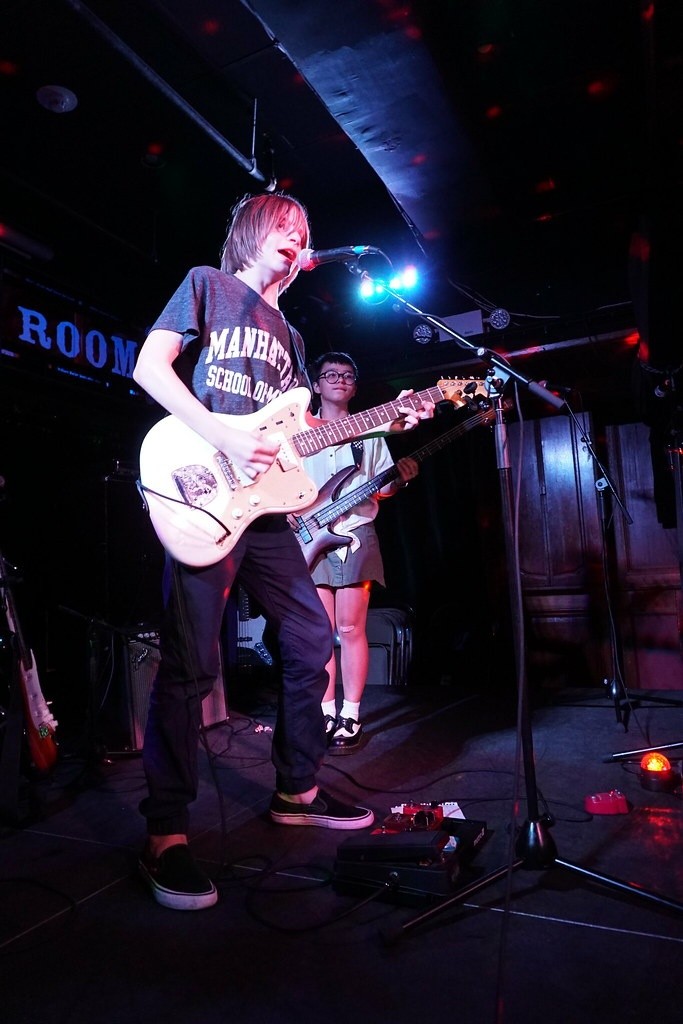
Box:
[296,246,379,272]
[500,380,575,394]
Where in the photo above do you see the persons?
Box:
[132,194,435,909]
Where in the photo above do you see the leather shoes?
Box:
[324,715,337,739]
[329,715,366,754]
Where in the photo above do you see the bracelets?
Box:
[393,477,409,489]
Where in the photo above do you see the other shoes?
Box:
[138,839,218,910]
[270,787,374,830]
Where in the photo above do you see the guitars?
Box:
[0,545,65,776]
[237,581,275,678]
[287,396,516,572]
[138,376,504,568]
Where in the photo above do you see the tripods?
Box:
[50,604,165,786]
[342,251,683,953]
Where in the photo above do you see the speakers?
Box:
[96,623,229,757]
[102,475,240,714]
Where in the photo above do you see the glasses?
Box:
[318,371,357,385]
